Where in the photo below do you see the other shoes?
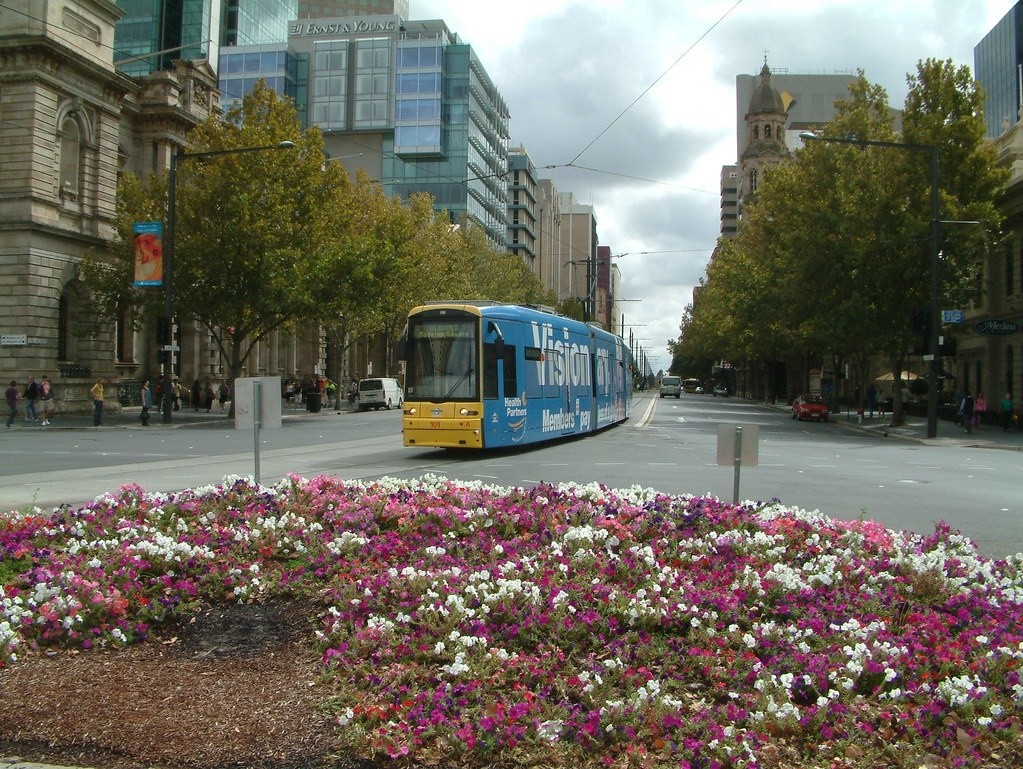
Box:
[98,423,104,426]
[45,419,50,424]
[24,418,31,421]
[34,419,39,422]
[42,421,45,426]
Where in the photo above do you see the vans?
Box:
[357,378,404,411]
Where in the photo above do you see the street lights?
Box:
[798,131,938,438]
[163,139,298,424]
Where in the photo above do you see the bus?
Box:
[684,378,700,394]
[396,257,634,449]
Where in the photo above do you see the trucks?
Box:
[659,376,682,399]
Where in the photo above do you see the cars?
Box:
[695,386,705,395]
[713,386,728,397]
[792,393,829,423]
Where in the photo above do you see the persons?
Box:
[996,392,1015,432]
[22,374,39,422]
[141,379,153,426]
[866,382,887,418]
[91,377,104,426]
[190,380,203,412]
[204,382,213,413]
[159,371,173,423]
[5,379,21,429]
[636,381,644,392]
[348,377,358,404]
[959,390,976,433]
[853,384,860,406]
[37,375,55,426]
[155,375,164,414]
[976,393,987,428]
[172,378,181,410]
[901,383,910,418]
[217,379,230,414]
[284,374,337,411]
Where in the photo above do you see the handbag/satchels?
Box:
[970,416,977,426]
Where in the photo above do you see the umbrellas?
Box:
[874,368,925,380]
[920,368,956,380]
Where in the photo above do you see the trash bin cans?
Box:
[306,393,322,413]
[347,393,355,404]
